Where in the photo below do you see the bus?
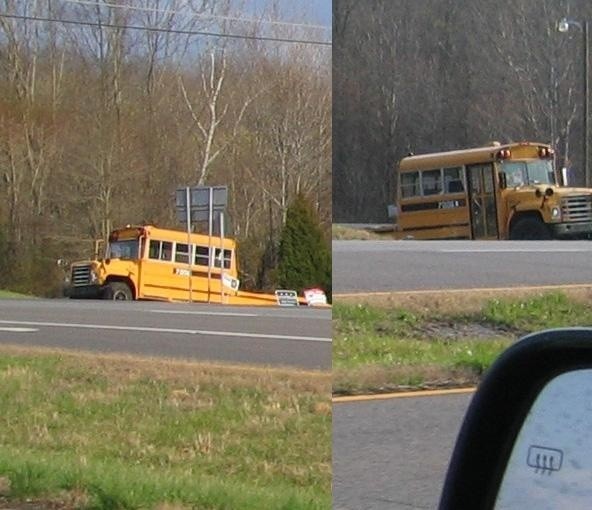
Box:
[392,134,592,241]
[62,224,242,308]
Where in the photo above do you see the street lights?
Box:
[554,14,592,186]
